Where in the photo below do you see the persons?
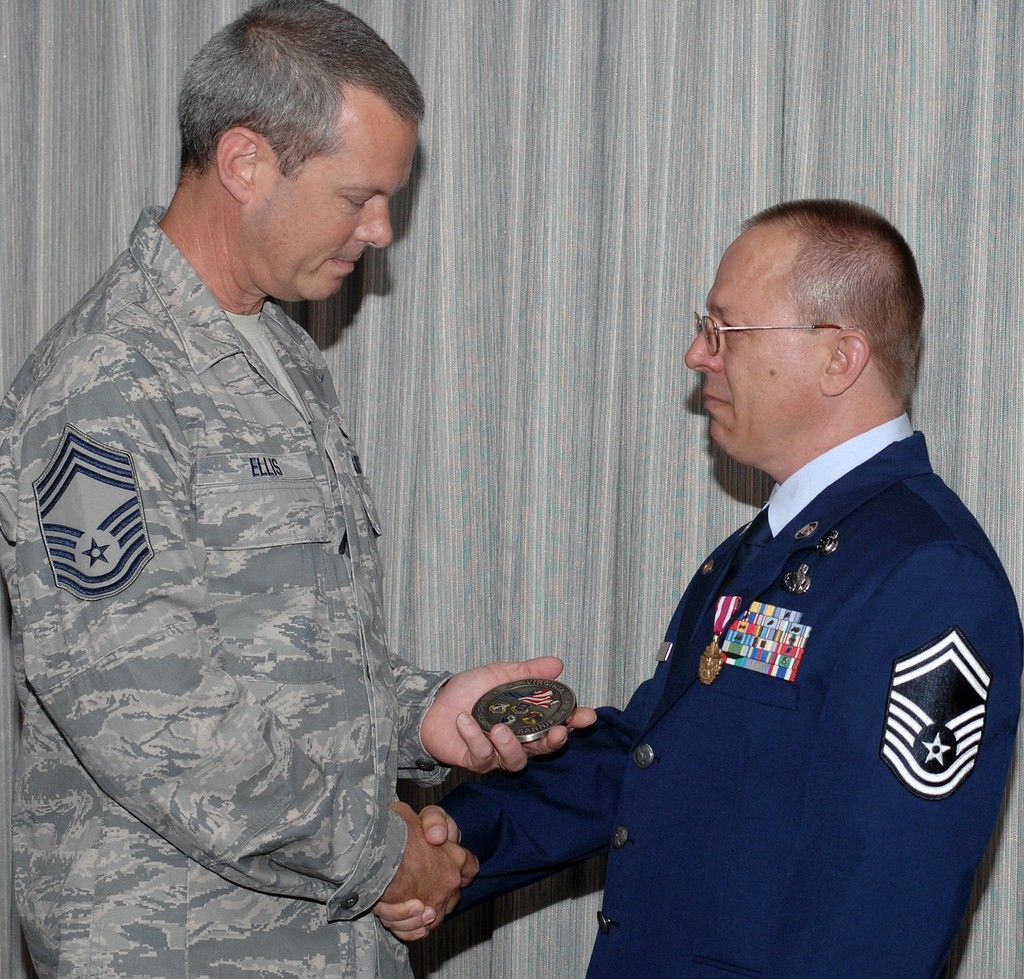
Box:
[2,2,596,978]
[373,199,1024,979]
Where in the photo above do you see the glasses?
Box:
[694,311,844,356]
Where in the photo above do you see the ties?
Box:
[704,505,772,619]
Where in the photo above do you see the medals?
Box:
[470,678,577,744]
